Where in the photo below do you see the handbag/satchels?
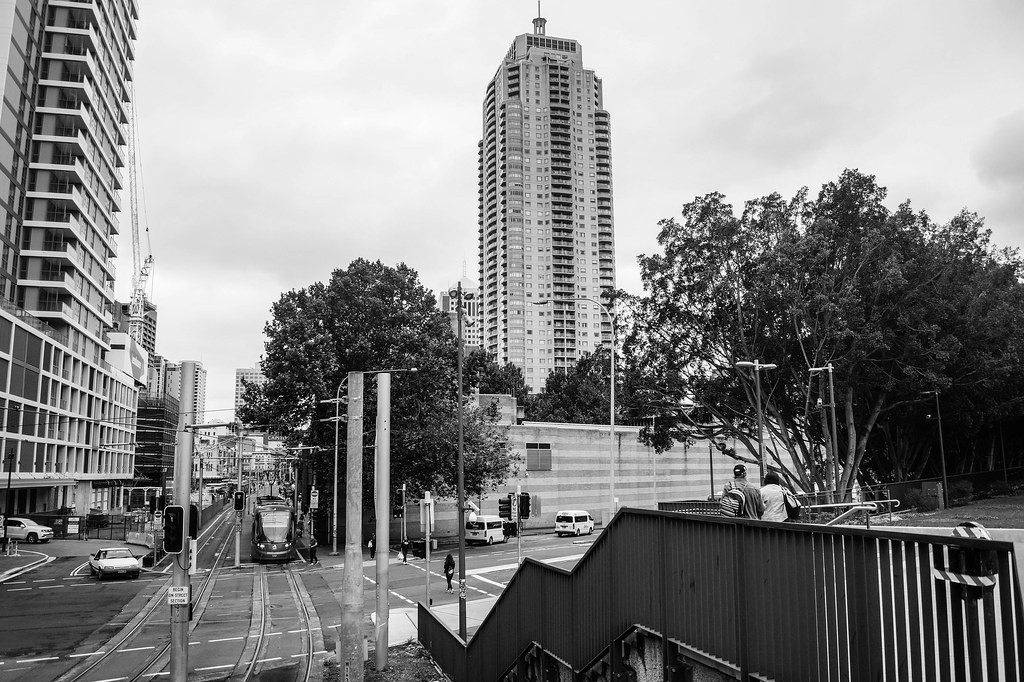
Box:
[779,484,801,520]
[368,541,372,548]
[398,553,404,559]
[448,569,454,574]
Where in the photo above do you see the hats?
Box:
[734,465,747,475]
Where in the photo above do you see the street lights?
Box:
[920,390,949,508]
[736,359,778,488]
[808,363,842,517]
[531,297,616,524]
[328,367,419,557]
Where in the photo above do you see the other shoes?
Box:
[451,589,454,594]
[315,561,319,565]
[403,562,406,564]
[308,563,314,566]
[405,562,409,565]
[445,588,450,593]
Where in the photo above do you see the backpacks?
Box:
[720,482,745,517]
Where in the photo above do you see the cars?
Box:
[189,474,255,509]
[0,517,55,544]
[88,548,141,582]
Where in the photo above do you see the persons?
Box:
[369,532,376,559]
[444,554,455,594]
[759,472,788,522]
[309,535,319,565]
[721,465,765,521]
[401,536,410,565]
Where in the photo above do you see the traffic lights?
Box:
[234,491,245,512]
[162,505,185,555]
[395,490,402,506]
[411,539,426,559]
[499,494,513,520]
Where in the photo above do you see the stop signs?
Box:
[154,511,162,519]
[312,490,319,498]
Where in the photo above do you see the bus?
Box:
[250,469,298,564]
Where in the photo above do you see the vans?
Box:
[554,510,595,537]
[465,515,510,546]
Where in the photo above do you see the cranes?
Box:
[124,61,154,346]
